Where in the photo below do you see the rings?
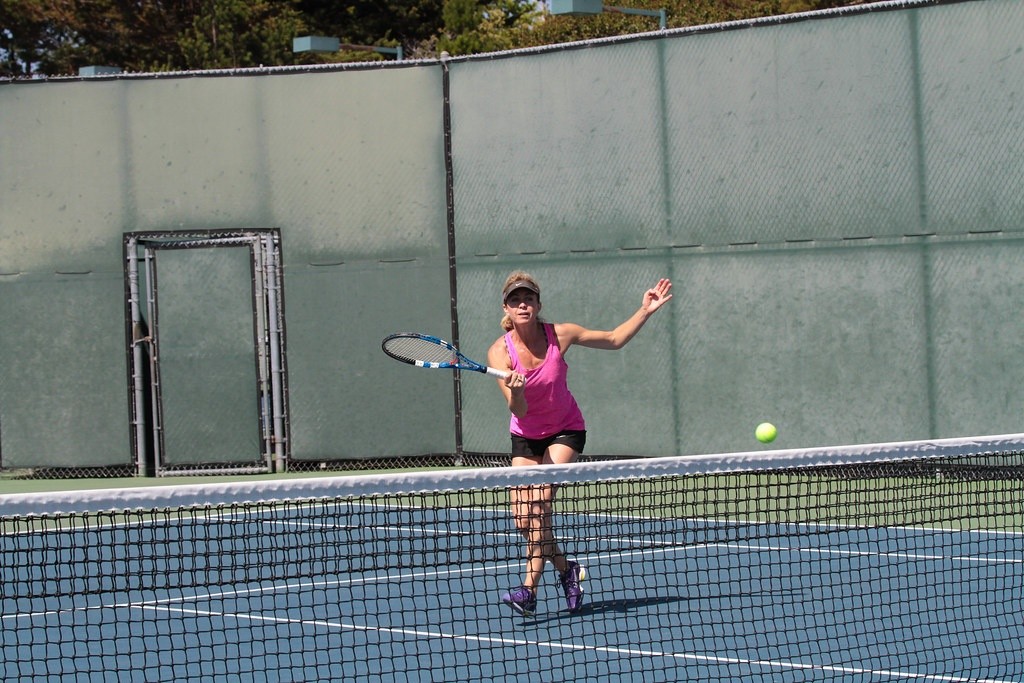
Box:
[518,378,522,382]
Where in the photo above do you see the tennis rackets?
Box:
[382,332,526,385]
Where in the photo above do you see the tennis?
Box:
[756,422,778,443]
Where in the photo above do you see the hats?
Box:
[502,278,540,304]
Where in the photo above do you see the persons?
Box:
[487,272,673,619]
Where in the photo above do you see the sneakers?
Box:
[503,585,538,619]
[555,561,586,615]
